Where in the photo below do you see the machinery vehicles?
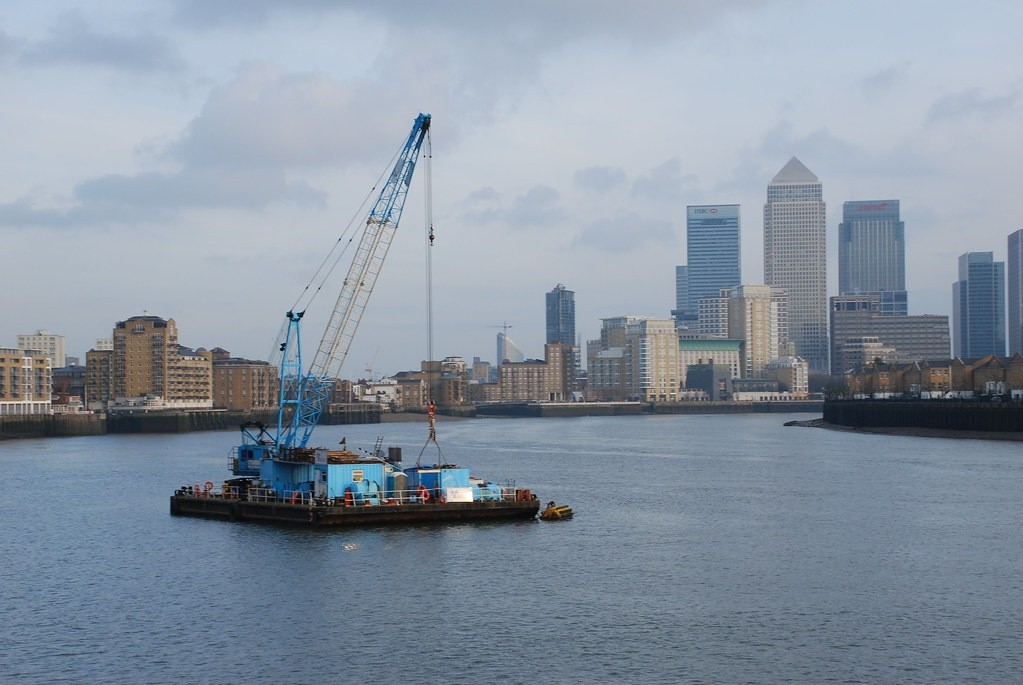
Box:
[223,108,431,501]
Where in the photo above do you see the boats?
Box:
[170,477,543,530]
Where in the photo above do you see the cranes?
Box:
[364,345,381,381]
[485,320,512,359]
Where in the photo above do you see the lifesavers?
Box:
[420,490,430,501]
[417,485,425,494]
[441,494,447,503]
[204,482,214,490]
